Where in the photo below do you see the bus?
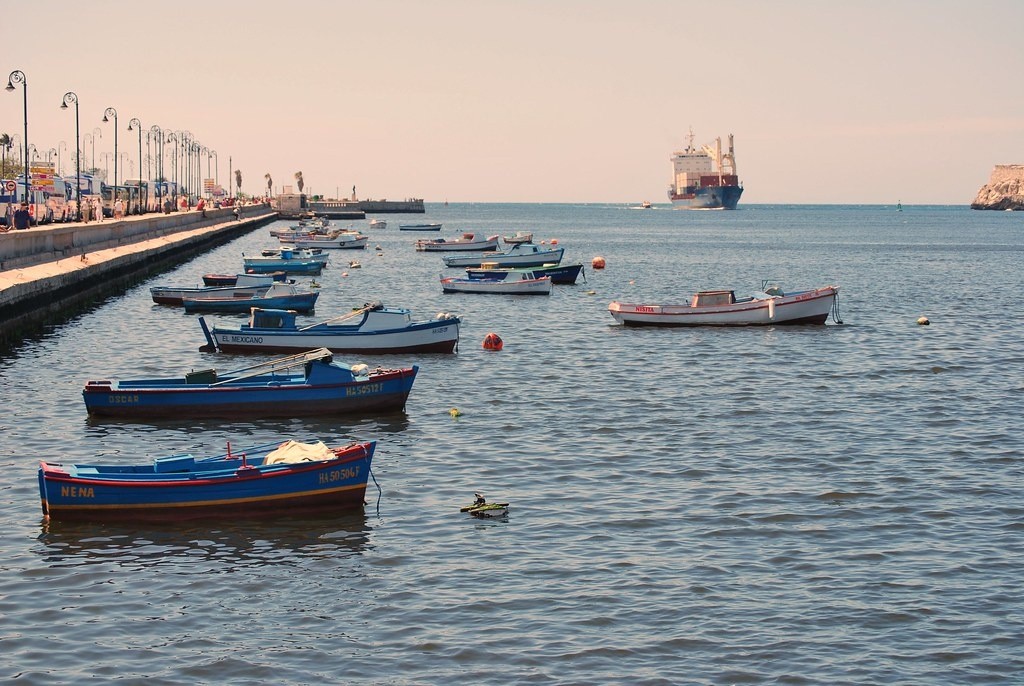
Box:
[0,174,184,228]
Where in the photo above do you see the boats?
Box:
[202,271,297,286]
[181,283,320,315]
[438,274,551,296]
[465,260,584,285]
[240,246,329,277]
[149,274,310,305]
[399,223,442,232]
[608,286,840,325]
[414,233,500,252]
[269,218,369,250]
[442,242,565,268]
[82,347,420,415]
[643,201,651,208]
[369,220,387,229]
[199,299,464,354]
[36,438,376,516]
[503,231,534,244]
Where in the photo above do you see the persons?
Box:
[114,198,121,221]
[81,198,91,224]
[4,203,13,226]
[12,202,32,230]
[1,193,270,230]
[196,200,208,218]
[164,197,172,215]
[233,208,241,220]
[94,197,104,223]
[120,199,125,218]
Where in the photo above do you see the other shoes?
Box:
[202,215,207,218]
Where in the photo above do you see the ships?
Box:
[667,125,744,210]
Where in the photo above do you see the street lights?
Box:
[4,69,218,227]
